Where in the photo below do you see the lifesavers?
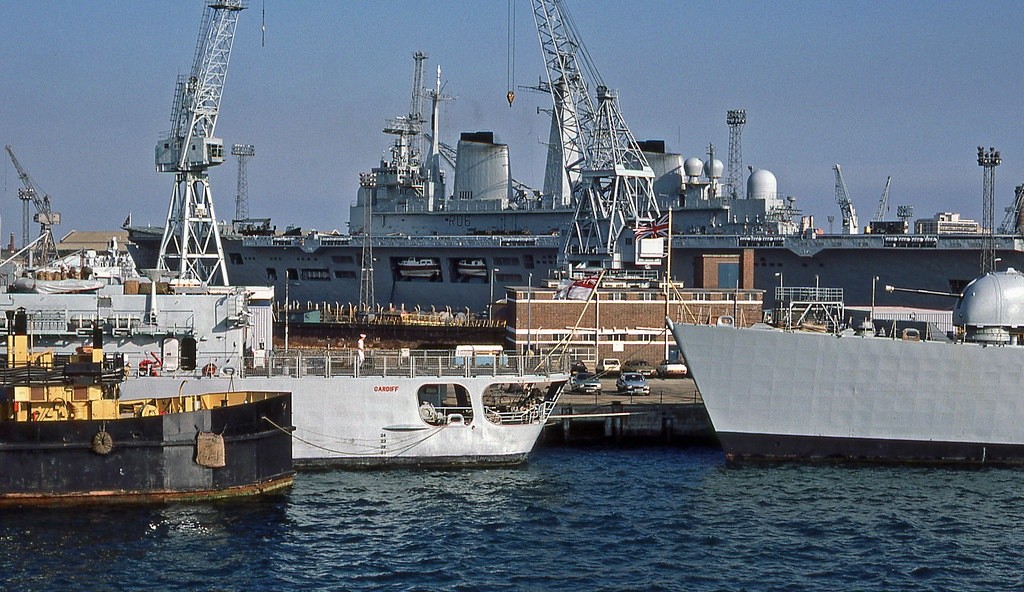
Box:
[205,362,216,375]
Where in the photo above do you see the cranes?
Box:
[493,0,676,299]
[149,0,267,284]
[3,144,61,227]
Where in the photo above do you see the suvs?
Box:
[595,357,620,376]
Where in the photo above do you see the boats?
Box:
[666,266,1023,466]
[456,259,488,278]
[0,391,298,511]
[0,307,574,473]
[397,258,440,280]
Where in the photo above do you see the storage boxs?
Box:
[124,281,139,295]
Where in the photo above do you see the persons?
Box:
[357,333,366,369]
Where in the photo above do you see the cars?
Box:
[656,358,686,378]
[621,360,657,378]
[572,373,602,396]
[570,359,587,375]
[614,373,650,396]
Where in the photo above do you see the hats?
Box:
[360,334,366,338]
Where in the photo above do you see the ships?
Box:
[118,53,1023,334]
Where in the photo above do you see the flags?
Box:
[633,213,669,241]
[555,274,599,301]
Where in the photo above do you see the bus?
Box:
[456,345,508,369]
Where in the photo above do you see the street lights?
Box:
[488,269,497,323]
[870,276,879,327]
[815,275,820,314]
[774,271,784,323]
[528,272,532,356]
